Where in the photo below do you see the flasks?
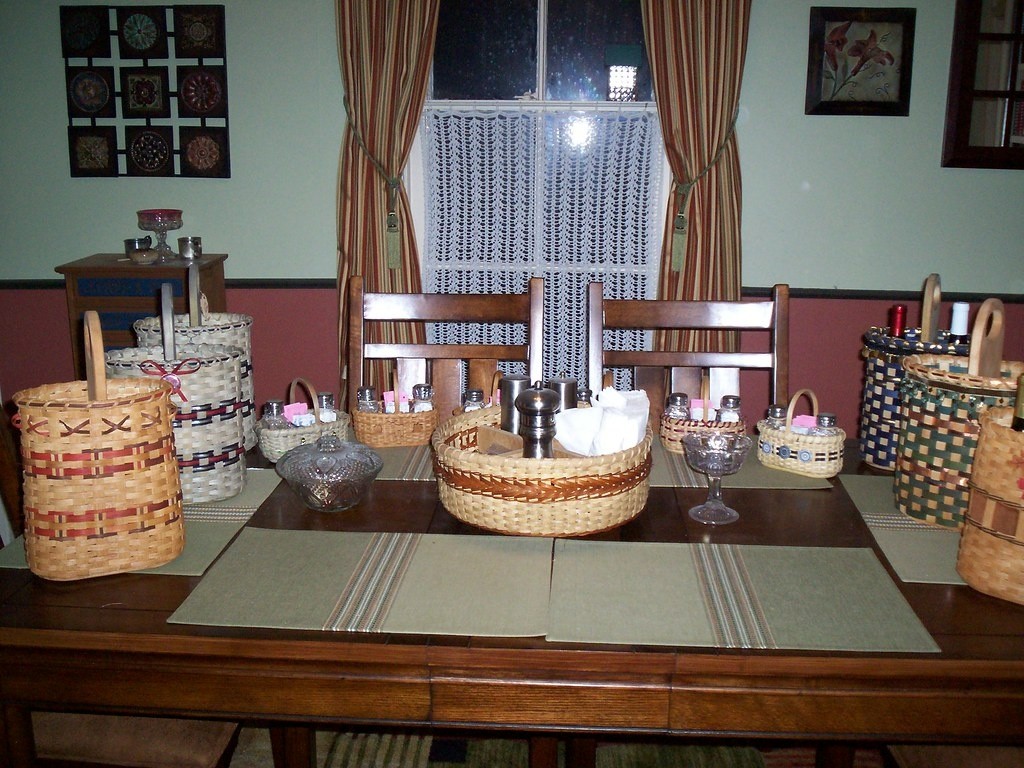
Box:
[550,378,577,414]
[500,375,530,435]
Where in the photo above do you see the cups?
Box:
[124,235,153,258]
[178,237,202,260]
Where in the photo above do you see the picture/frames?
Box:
[805,6,916,116]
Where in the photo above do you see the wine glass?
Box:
[680,432,752,526]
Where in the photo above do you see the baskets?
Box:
[133,263,258,451]
[452,372,503,417]
[254,376,351,463]
[352,401,438,448]
[756,389,847,478]
[432,406,653,538]
[103,283,246,505]
[13,312,186,580]
[955,406,1024,606]
[658,410,746,454]
[893,353,1024,530]
[857,272,972,471]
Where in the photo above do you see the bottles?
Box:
[259,400,291,430]
[357,386,378,414]
[663,393,691,421]
[316,391,339,424]
[461,388,485,414]
[716,395,742,422]
[765,404,788,430]
[809,413,838,437]
[515,380,561,459]
[577,389,592,409]
[888,304,907,340]
[411,384,433,413]
[948,302,970,345]
[1011,373,1024,433]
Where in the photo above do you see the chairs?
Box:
[348,275,545,427]
[588,282,789,435]
[0,398,310,768]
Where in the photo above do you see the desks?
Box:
[0,427,1024,768]
[53,252,228,380]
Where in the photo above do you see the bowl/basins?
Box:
[275,435,384,513]
[130,248,159,265]
